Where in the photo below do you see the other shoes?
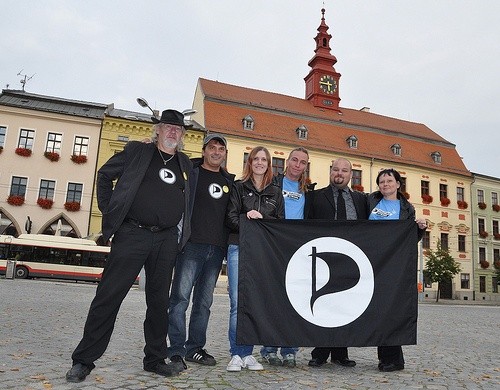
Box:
[308,358,324,366]
[334,357,356,367]
[378,362,405,371]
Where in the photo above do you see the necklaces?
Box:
[157,146,174,166]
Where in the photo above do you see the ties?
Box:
[337,189,346,221]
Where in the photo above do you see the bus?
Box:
[0,233,141,290]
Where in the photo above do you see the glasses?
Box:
[165,124,183,134]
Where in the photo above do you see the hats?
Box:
[204,135,227,148]
[160,110,184,126]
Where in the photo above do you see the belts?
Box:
[129,219,171,232]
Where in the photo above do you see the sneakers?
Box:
[241,355,264,370]
[66,363,91,383]
[226,354,246,371]
[185,348,216,366]
[262,353,283,367]
[283,353,296,368]
[170,355,187,370]
[148,362,179,377]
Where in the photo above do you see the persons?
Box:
[167,133,236,370]
[226,146,285,371]
[307,157,365,368]
[259,147,317,367]
[65,109,200,382]
[353,169,427,372]
